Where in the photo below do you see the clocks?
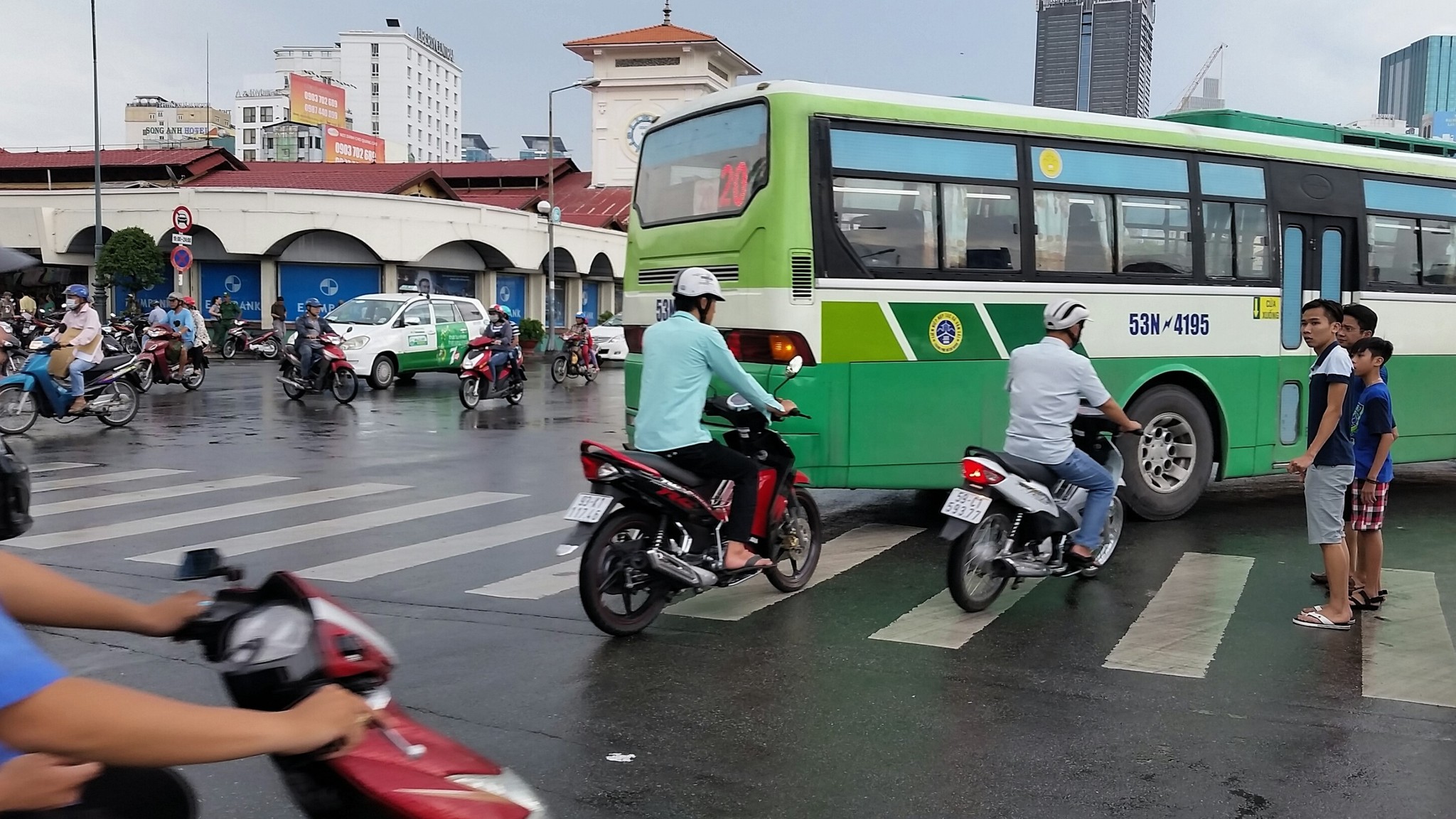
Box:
[625,111,655,152]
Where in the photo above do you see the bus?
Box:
[622,78,1456,521]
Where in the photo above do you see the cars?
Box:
[285,284,491,389]
[589,311,630,367]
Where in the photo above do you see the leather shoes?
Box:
[1310,569,1365,593]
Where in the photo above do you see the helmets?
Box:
[150,299,160,308]
[671,267,727,302]
[1043,297,1094,330]
[575,311,589,324]
[305,298,324,308]
[486,304,512,316]
[4,291,13,296]
[166,292,195,307]
[61,284,89,298]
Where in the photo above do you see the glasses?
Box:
[1337,327,1365,334]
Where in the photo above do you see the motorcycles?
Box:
[276,327,359,404]
[552,332,604,383]
[221,318,282,360]
[0,546,550,819]
[938,397,1145,612]
[0,323,147,435]
[458,327,528,409]
[556,355,822,635]
[0,303,150,376]
[126,320,210,394]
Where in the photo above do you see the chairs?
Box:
[376,307,390,323]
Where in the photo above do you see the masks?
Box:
[489,315,500,322]
[66,299,80,310]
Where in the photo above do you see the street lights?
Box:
[537,77,601,351]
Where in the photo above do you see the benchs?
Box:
[847,202,1103,269]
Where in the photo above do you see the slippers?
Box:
[1293,605,1356,630]
[68,401,88,413]
[725,554,778,575]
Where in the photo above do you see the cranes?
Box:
[1161,42,1225,113]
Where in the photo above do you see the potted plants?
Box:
[517,317,548,349]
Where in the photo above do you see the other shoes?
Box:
[212,344,217,348]
[194,370,201,374]
[514,384,521,390]
[215,348,221,353]
[491,388,497,393]
[300,380,313,389]
[174,375,184,380]
[1068,552,1096,566]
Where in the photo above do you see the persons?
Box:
[141,299,168,352]
[0,537,405,819]
[1346,336,1399,611]
[44,294,55,318]
[209,292,243,348]
[125,292,143,320]
[1284,298,1390,630]
[561,312,600,375]
[19,289,39,321]
[0,291,16,319]
[60,282,105,413]
[162,291,211,381]
[631,265,783,580]
[270,295,288,340]
[293,293,334,388]
[419,278,431,294]
[482,304,521,389]
[1003,295,1143,570]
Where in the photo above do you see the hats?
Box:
[277,296,284,301]
[223,292,231,297]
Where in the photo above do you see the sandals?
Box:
[1346,586,1387,611]
